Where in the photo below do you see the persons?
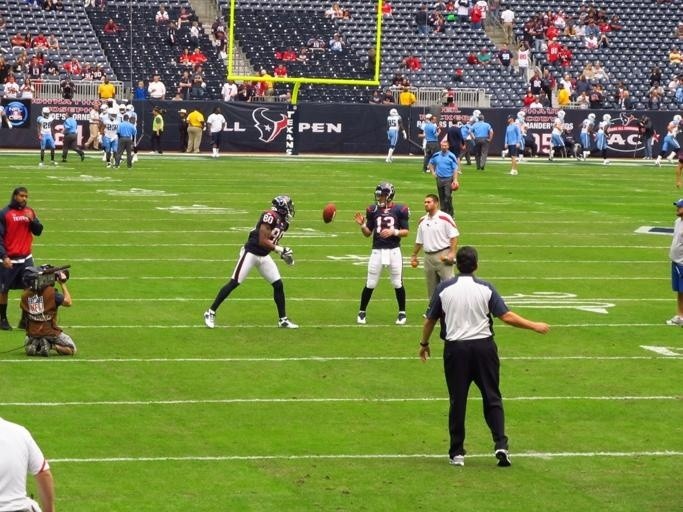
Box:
[104,17,119,33]
[221,49,306,102]
[381,1,393,17]
[352,181,410,325]
[0,32,116,100]
[156,7,169,25]
[369,43,513,107]
[168,8,209,101]
[36,1,107,14]
[211,14,230,61]
[383,107,681,175]
[414,1,487,34]
[664,198,683,330]
[18,265,76,357]
[427,141,460,219]
[37,101,138,169]
[0,186,42,331]
[150,108,164,154]
[307,32,345,52]
[410,194,459,320]
[135,75,166,101]
[178,106,227,156]
[0,414,54,512]
[325,3,352,22]
[203,194,301,329]
[418,245,551,465]
[498,3,680,111]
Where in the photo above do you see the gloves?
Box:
[281,247,294,266]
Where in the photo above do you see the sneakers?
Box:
[27,338,49,357]
[357,312,366,324]
[666,316,683,325]
[495,449,511,466]
[203,308,215,329]
[278,319,299,328]
[450,455,464,466]
[0,315,12,329]
[396,313,406,325]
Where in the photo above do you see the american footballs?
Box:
[322,202,336,224]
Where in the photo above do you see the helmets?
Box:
[100,104,134,120]
[673,114,682,122]
[588,113,611,120]
[517,111,525,119]
[41,107,50,118]
[425,114,432,119]
[473,110,481,117]
[271,196,295,223]
[374,183,395,207]
[557,110,566,118]
[389,108,398,115]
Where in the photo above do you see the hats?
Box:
[178,109,187,114]
[673,199,683,207]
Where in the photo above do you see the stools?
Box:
[0,2,683,111]
